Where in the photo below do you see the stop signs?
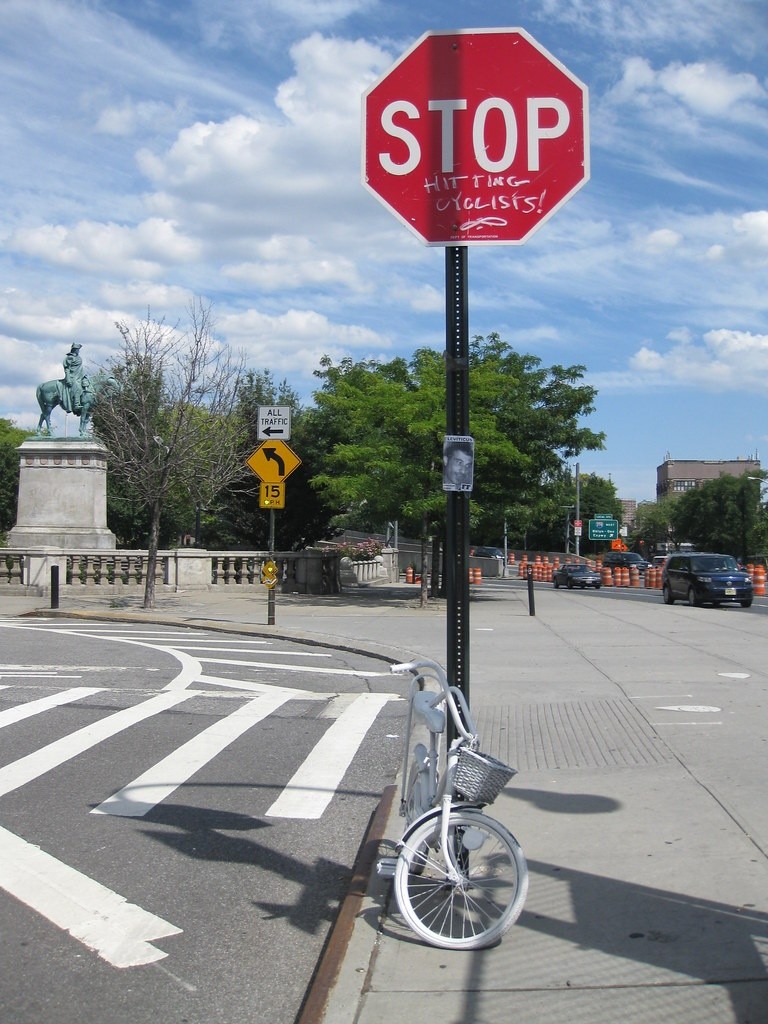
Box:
[364,26,586,248]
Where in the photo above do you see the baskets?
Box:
[453,747,518,804]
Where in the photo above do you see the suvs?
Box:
[603,551,653,573]
[661,552,753,608]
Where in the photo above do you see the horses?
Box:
[36,374,122,436]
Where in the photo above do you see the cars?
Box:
[553,564,603,589]
[474,547,508,566]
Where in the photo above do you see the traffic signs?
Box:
[588,514,619,541]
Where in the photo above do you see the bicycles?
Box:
[384,658,527,951]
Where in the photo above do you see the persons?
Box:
[63,342,91,417]
[186,533,191,546]
[443,442,473,485]
